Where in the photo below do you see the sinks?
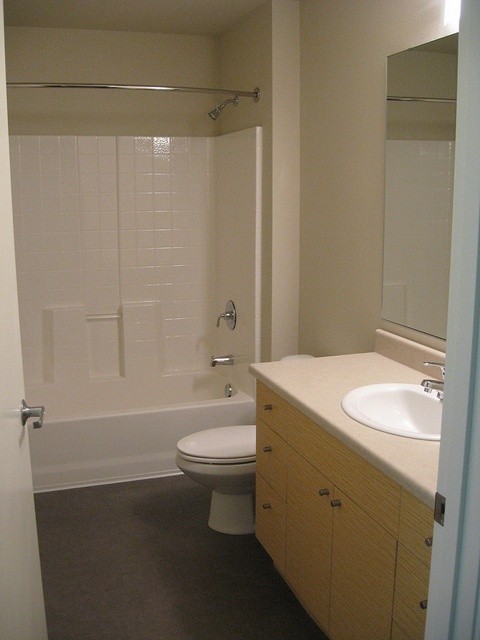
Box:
[341,382,445,442]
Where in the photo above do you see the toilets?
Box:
[174,425,256,535]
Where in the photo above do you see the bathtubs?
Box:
[24,369,256,495]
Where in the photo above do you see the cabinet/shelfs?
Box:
[255,378,438,640]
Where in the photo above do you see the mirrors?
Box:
[382,33,459,338]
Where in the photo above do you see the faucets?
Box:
[419,360,444,403]
[209,355,234,370]
